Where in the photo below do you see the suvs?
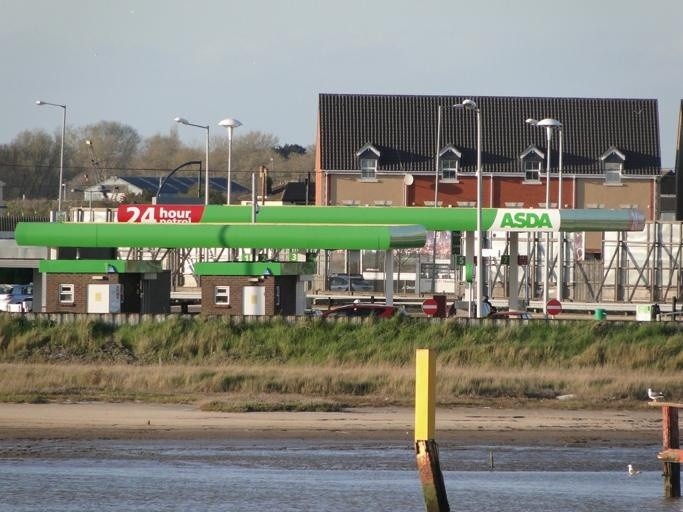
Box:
[328,272,376,294]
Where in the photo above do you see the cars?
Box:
[316,303,403,320]
[0,281,33,315]
[478,311,533,320]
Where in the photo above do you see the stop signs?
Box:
[546,299,564,315]
[421,298,437,315]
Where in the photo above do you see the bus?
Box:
[413,256,459,295]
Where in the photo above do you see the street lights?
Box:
[537,118,564,312]
[463,99,484,321]
[217,118,243,263]
[526,116,553,299]
[174,116,211,263]
[34,100,68,210]
[430,103,464,298]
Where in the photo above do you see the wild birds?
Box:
[648,388,665,402]
[626,464,642,477]
